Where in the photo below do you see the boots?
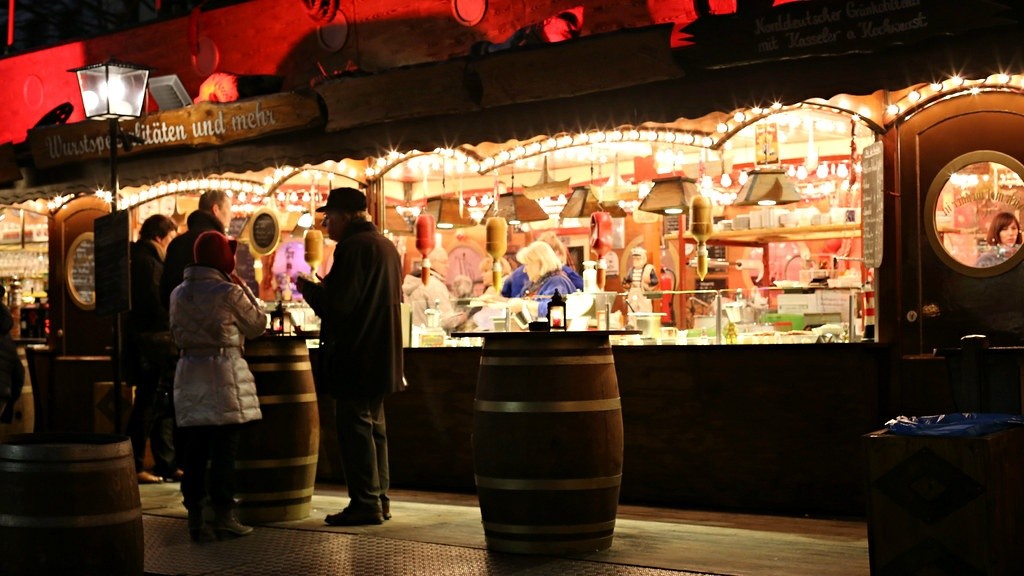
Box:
[214,508,254,542]
[187,508,213,542]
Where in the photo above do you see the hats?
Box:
[193,230,238,275]
[315,187,368,213]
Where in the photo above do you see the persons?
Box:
[297,188,409,528]
[167,232,270,547]
[501,231,583,298]
[160,191,241,301]
[401,247,470,333]
[976,213,1023,268]
[514,241,577,318]
[622,247,660,313]
[132,215,179,484]
[0,284,25,425]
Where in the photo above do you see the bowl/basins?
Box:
[686,331,819,344]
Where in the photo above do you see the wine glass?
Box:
[0,251,49,294]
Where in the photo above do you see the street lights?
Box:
[65,53,153,441]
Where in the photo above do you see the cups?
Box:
[636,314,679,345]
[72,241,95,302]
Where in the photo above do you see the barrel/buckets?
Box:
[0,432,144,576]
[473,334,624,557]
[239,339,319,524]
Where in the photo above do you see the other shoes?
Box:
[137,471,164,483]
[164,469,184,482]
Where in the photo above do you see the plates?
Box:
[772,280,808,288]
[717,207,861,230]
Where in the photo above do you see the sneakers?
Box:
[382,507,392,518]
[325,507,385,526]
